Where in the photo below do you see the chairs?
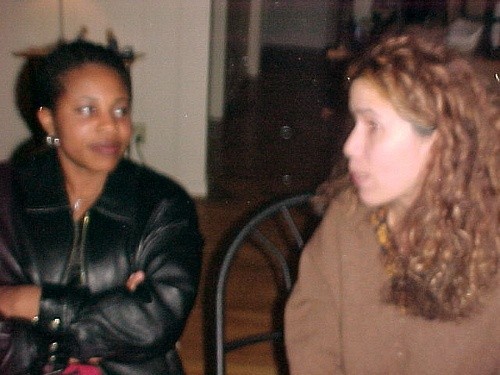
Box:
[204,189,318,375]
